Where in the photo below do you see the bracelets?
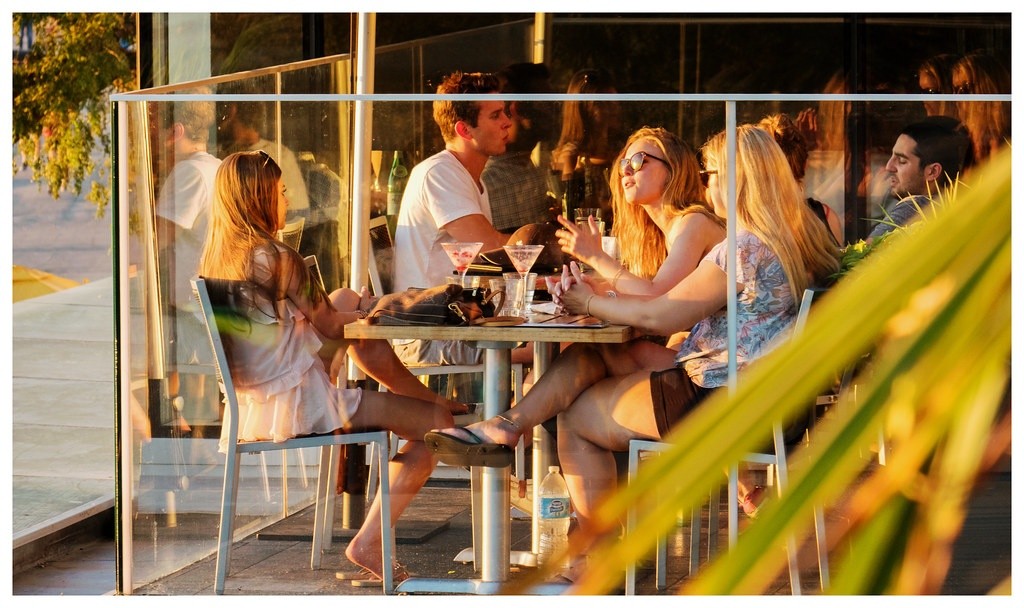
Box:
[586,294,597,316]
[608,265,628,297]
[355,310,366,317]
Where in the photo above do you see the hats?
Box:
[478,222,565,274]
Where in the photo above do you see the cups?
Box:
[502,272,538,316]
[573,207,606,235]
[445,276,481,296]
[489,279,524,317]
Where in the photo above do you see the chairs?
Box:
[187,214,890,593]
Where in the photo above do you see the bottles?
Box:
[386,150,409,238]
[537,465,571,582]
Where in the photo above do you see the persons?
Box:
[150,86,313,495]
[199,49,1013,588]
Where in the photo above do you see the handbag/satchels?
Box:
[366,283,524,327]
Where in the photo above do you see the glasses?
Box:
[619,151,671,177]
[259,150,275,170]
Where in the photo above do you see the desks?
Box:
[344,313,643,595]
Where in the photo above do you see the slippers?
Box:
[336,568,377,580]
[352,564,410,587]
[424,426,515,468]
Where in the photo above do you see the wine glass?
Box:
[501,244,544,321]
[440,242,484,288]
